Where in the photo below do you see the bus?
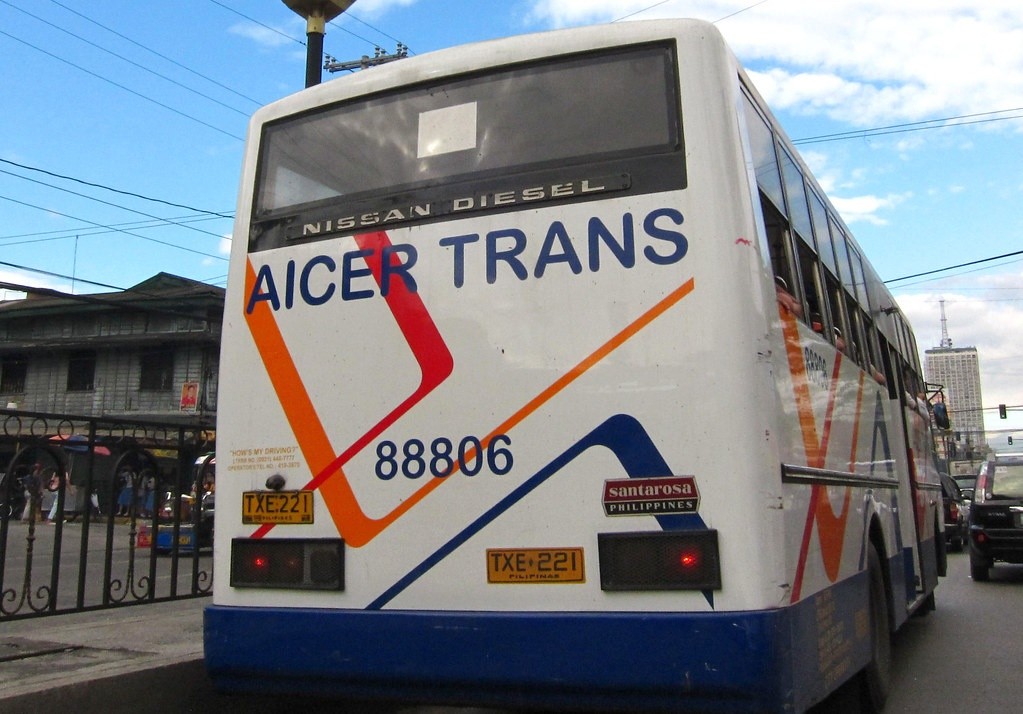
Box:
[203,12,952,714]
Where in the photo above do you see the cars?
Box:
[938,471,970,553]
[951,475,978,498]
[968,452,1023,581]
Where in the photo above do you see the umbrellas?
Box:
[48,434,111,480]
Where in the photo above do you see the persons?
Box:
[775,284,930,420]
[189,480,215,511]
[9,461,75,525]
[114,466,156,518]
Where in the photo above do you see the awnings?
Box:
[84,414,217,431]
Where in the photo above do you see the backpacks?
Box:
[48,476,60,491]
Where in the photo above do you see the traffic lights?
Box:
[1008,436,1012,446]
[1000,405,1007,419]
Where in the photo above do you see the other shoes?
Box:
[21,519,30,524]
[124,512,129,516]
[147,514,152,519]
[49,519,67,526]
[115,512,123,517]
[140,514,145,518]
